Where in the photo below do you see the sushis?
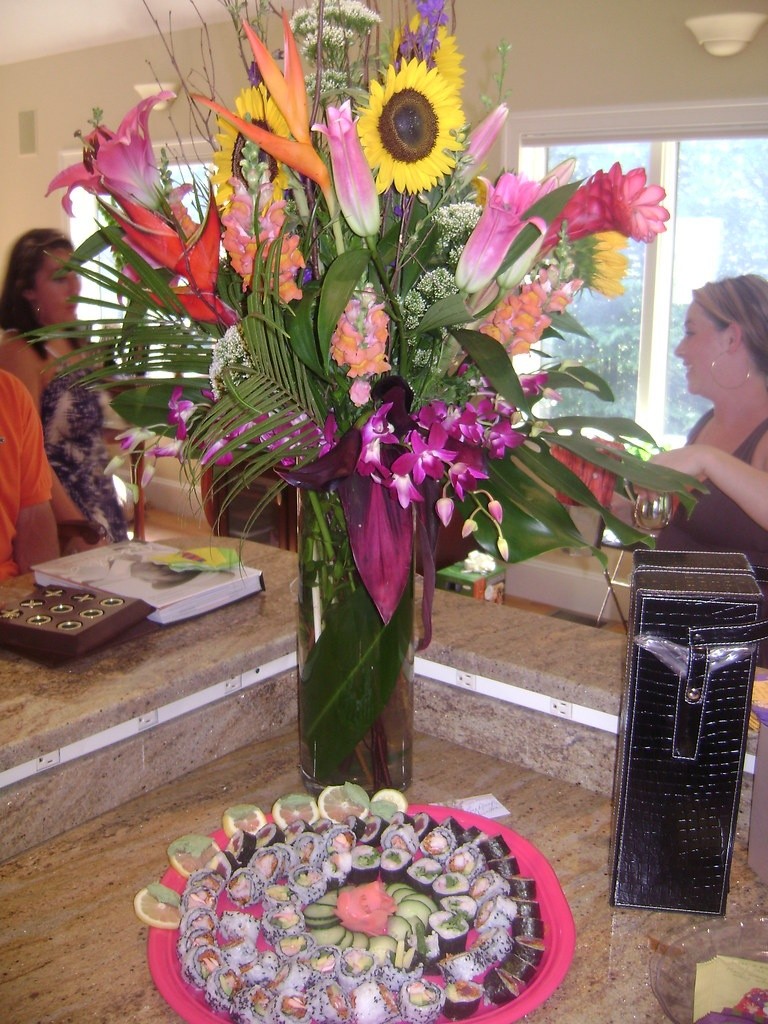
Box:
[177,811,546,1024]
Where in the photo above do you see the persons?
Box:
[46,547,202,590]
[0,228,145,555]
[635,274,768,669]
[0,368,60,585]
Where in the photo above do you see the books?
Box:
[29,540,266,629]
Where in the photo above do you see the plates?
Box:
[146,805,577,1024]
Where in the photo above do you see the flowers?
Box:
[335,878,397,938]
[3,0,713,651]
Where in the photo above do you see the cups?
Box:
[635,490,673,529]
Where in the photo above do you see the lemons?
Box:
[134,782,409,930]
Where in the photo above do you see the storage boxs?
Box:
[609,572,762,919]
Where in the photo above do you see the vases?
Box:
[297,503,413,796]
